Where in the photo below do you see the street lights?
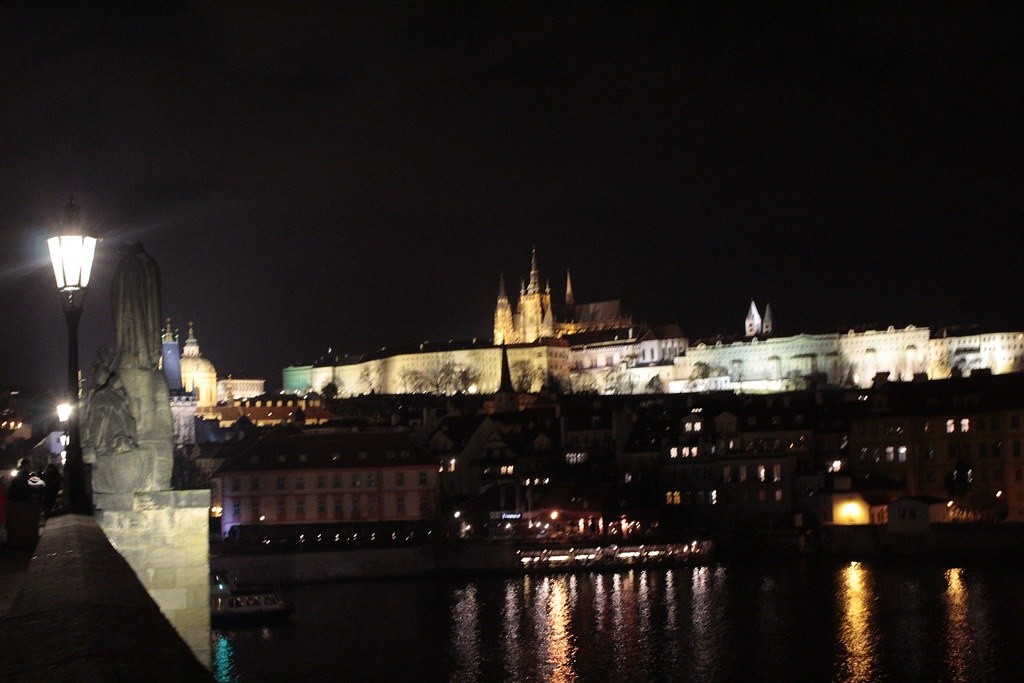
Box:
[43,219,105,511]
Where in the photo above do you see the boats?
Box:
[203,569,292,627]
